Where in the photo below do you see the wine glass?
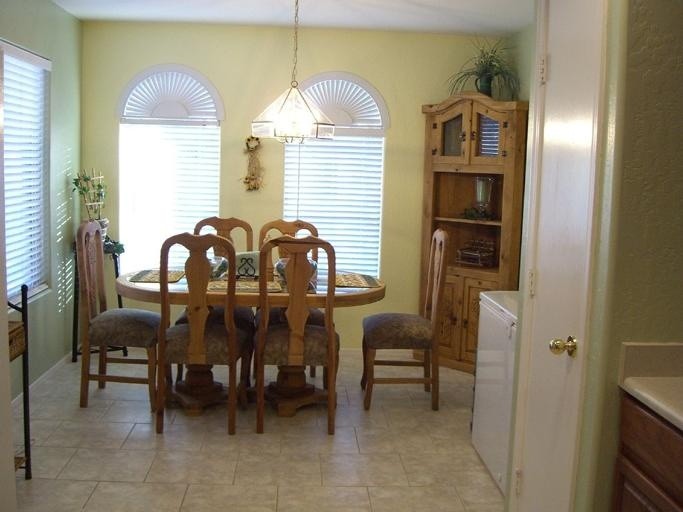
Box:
[473,176,494,219]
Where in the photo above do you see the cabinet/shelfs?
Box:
[412,90,529,375]
[6,283,32,480]
[608,393,682,511]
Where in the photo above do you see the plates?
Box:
[478,113,500,157]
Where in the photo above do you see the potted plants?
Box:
[441,33,520,99]
[70,167,110,247]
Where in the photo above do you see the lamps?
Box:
[250,1,335,147]
